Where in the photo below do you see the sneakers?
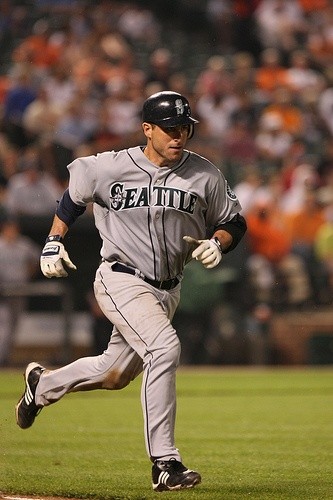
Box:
[151,457,202,492]
[15,362,48,429]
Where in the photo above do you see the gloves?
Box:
[182,235,224,269]
[39,235,77,279]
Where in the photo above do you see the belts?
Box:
[112,262,180,290]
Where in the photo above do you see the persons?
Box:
[0,0,333,367]
[16,91,248,493]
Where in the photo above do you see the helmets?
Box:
[142,91,200,139]
